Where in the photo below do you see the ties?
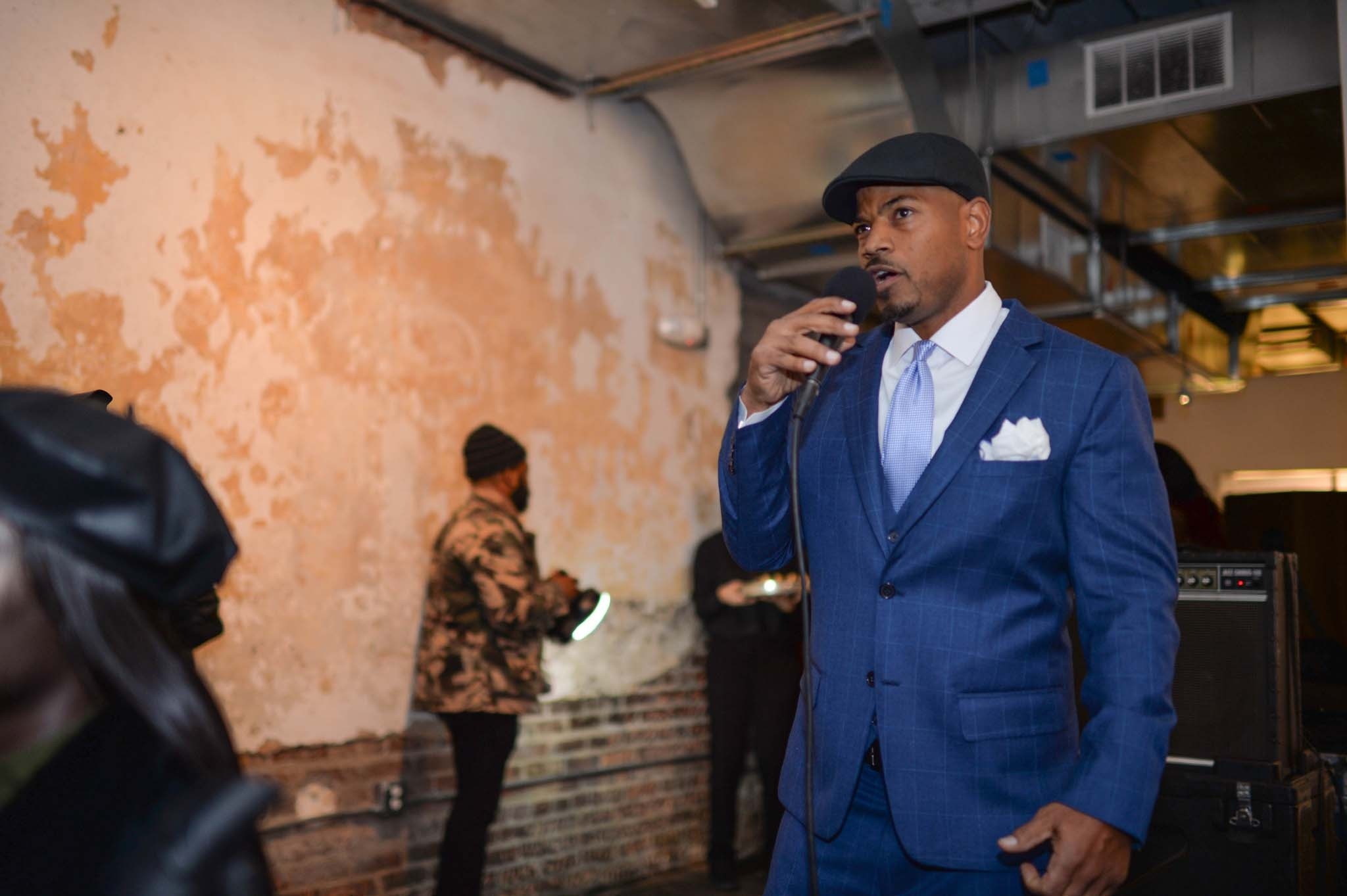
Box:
[882,340,937,514]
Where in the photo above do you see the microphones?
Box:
[793,266,877,422]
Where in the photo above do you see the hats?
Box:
[0,383,241,645]
[822,133,989,225]
[464,426,525,479]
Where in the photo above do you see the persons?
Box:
[412,423,580,896]
[695,523,814,891]
[0,377,278,896]
[717,135,1183,896]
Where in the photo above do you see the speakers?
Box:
[1063,549,1303,786]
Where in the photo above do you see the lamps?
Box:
[557,571,610,641]
